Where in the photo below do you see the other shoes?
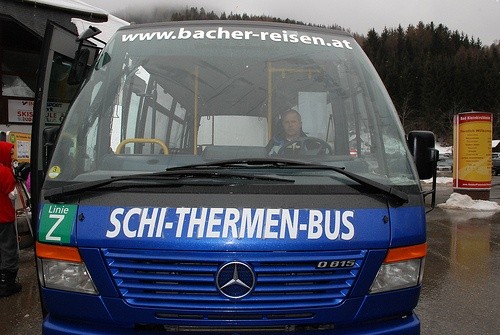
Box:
[0,269,23,297]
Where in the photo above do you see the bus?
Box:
[29,18,440,334]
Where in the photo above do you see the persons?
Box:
[266,110,316,157]
[0,141,22,296]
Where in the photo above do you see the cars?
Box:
[436,146,452,171]
[492,139,500,176]
